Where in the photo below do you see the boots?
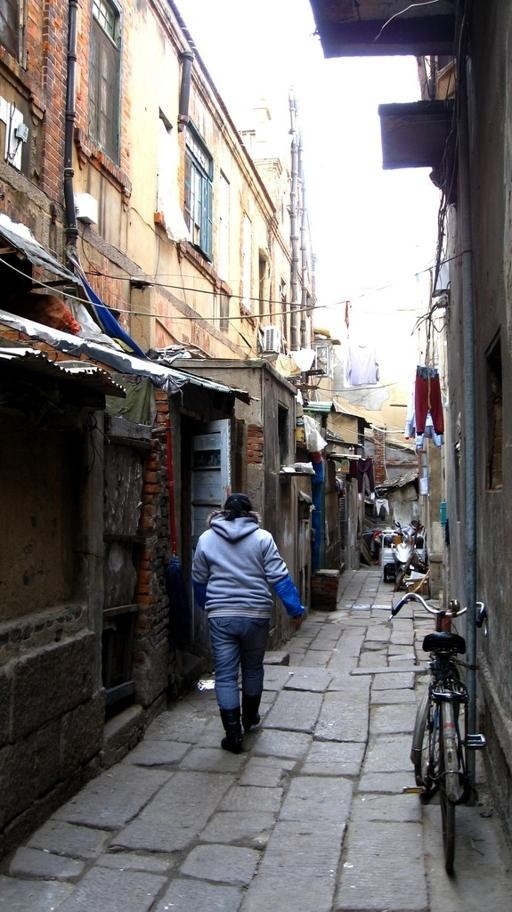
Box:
[219,692,262,754]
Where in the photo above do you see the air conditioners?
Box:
[263,325,283,355]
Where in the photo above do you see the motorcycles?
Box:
[382,520,428,592]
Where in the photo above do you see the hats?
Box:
[224,493,253,510]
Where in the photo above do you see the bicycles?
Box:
[388,593,490,873]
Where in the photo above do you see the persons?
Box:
[191,493,304,754]
